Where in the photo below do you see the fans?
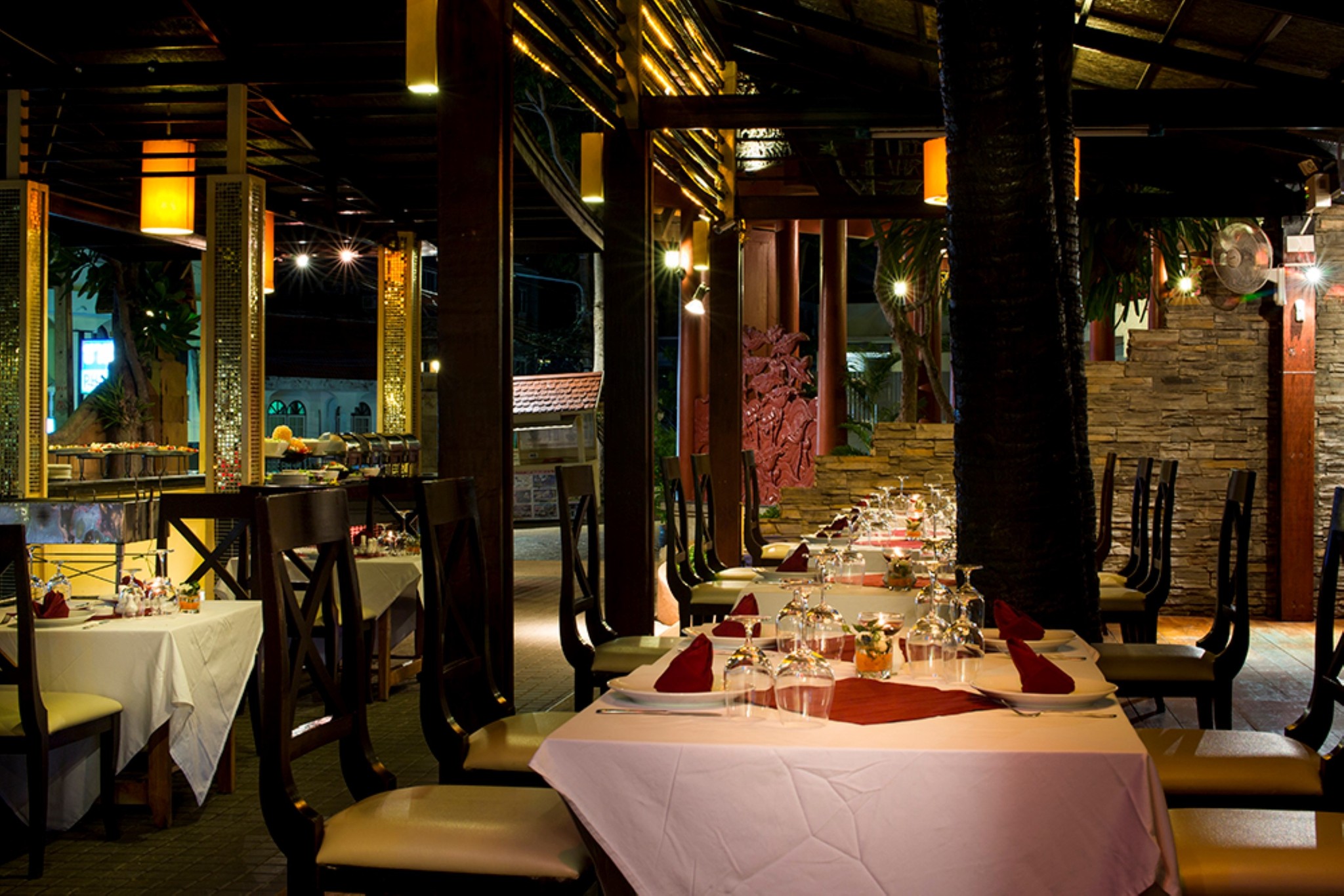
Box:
[1211,218,1287,306]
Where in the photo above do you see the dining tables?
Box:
[733,563,950,634]
[529,617,1183,896]
[218,549,425,700]
[0,598,265,830]
[787,538,952,574]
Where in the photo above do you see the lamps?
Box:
[582,132,604,203]
[923,136,948,206]
[685,283,711,314]
[406,0,439,95]
[140,105,197,235]
[693,221,710,270]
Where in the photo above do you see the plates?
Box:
[968,675,1118,711]
[14,610,97,627]
[982,629,1076,651]
[606,505,892,710]
[49,444,198,456]
[98,594,119,604]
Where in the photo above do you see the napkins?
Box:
[656,633,715,692]
[777,542,810,572]
[991,601,1046,640]
[712,592,761,637]
[1007,636,1075,696]
[32,591,70,619]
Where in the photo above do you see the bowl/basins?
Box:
[359,468,380,477]
[264,440,289,457]
[271,467,349,485]
[302,439,331,454]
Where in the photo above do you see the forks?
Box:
[996,644,1087,660]
[1001,699,1117,719]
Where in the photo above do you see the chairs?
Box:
[0,449,1344,896]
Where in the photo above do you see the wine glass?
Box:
[47,560,70,601]
[118,569,145,619]
[148,548,178,616]
[394,509,415,555]
[722,474,984,720]
[26,545,45,605]
[376,523,395,554]
